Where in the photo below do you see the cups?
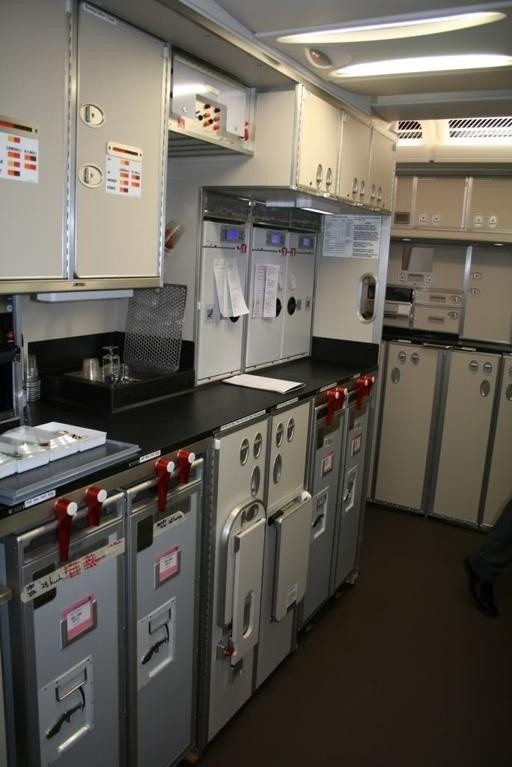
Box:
[165,220,184,252]
[23,354,40,402]
[81,357,101,382]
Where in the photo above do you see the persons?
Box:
[463,496,512,622]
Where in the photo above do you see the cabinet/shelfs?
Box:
[337,102,375,210]
[381,339,444,516]
[369,121,397,217]
[412,173,467,231]
[243,200,321,371]
[295,87,342,200]
[427,347,512,533]
[0,3,172,296]
[467,175,512,234]
[193,189,244,386]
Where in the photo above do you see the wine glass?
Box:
[102,346,121,381]
